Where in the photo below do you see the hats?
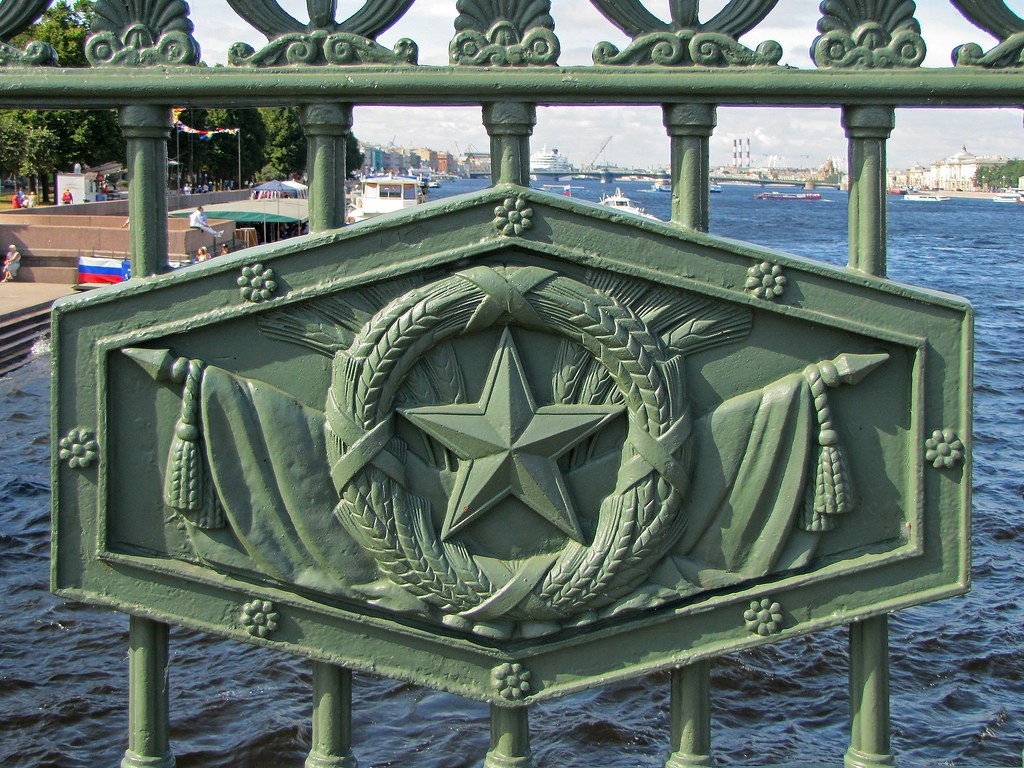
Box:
[9,245,16,250]
[222,244,228,248]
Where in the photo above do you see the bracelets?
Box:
[122,226,124,228]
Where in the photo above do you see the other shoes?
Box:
[219,230,224,235]
[6,275,14,280]
[215,235,221,238]
[2,279,8,283]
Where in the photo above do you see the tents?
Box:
[168,198,310,243]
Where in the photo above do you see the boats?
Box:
[653,179,723,193]
[903,193,950,202]
[755,192,822,200]
[992,193,1021,203]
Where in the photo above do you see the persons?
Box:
[121,217,129,228]
[184,179,254,196]
[12,180,118,209]
[1,245,21,282]
[190,205,232,263]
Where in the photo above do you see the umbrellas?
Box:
[254,180,297,192]
